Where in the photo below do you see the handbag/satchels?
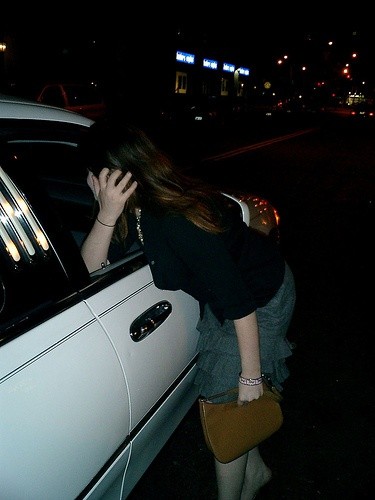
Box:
[197,375,284,464]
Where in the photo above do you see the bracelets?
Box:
[238,370,265,386]
[96,218,115,227]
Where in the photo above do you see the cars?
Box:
[1,94,283,500]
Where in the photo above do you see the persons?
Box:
[81,115,297,500]
